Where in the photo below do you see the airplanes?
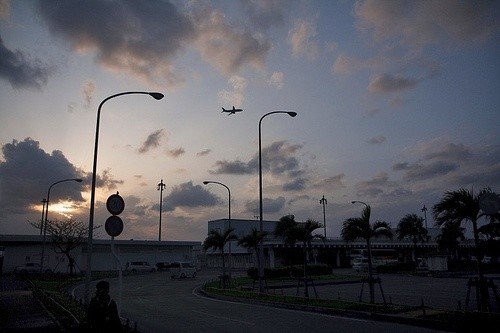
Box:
[221,106,244,116]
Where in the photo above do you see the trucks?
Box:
[414,254,448,277]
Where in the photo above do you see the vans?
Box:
[170,261,198,279]
[352,258,374,272]
[126,260,158,274]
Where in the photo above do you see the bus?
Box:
[349,248,399,268]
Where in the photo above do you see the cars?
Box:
[15,262,51,276]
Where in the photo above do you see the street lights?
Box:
[156,178,167,241]
[203,180,231,280]
[84,91,167,306]
[319,195,328,237]
[39,198,48,238]
[39,178,84,281]
[258,110,298,291]
[421,204,428,228]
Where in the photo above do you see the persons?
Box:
[86,280,125,333]
